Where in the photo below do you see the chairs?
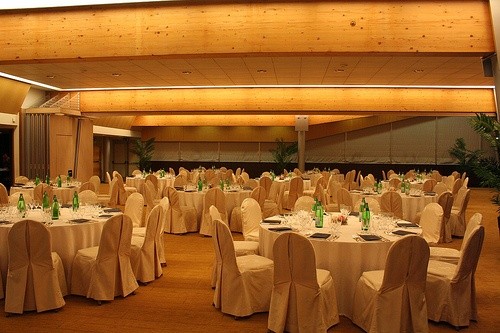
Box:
[0,160,488,333]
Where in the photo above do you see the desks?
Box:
[175,185,253,232]
[9,183,81,207]
[379,176,436,190]
[0,205,124,290]
[350,189,438,223]
[125,175,176,191]
[254,176,313,193]
[258,208,424,321]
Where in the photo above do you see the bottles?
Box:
[314,201,323,227]
[45,174,50,184]
[51,195,60,219]
[377,180,382,194]
[311,198,319,220]
[66,174,70,187]
[269,166,330,181]
[373,178,378,192]
[196,175,230,191]
[42,191,49,211]
[361,203,371,230]
[36,173,40,185]
[358,197,368,222]
[142,167,165,177]
[72,191,79,212]
[17,194,25,212]
[57,176,61,187]
[398,168,432,195]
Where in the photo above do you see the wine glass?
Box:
[279,203,397,242]
[25,177,82,188]
[385,184,402,196]
[0,199,103,227]
